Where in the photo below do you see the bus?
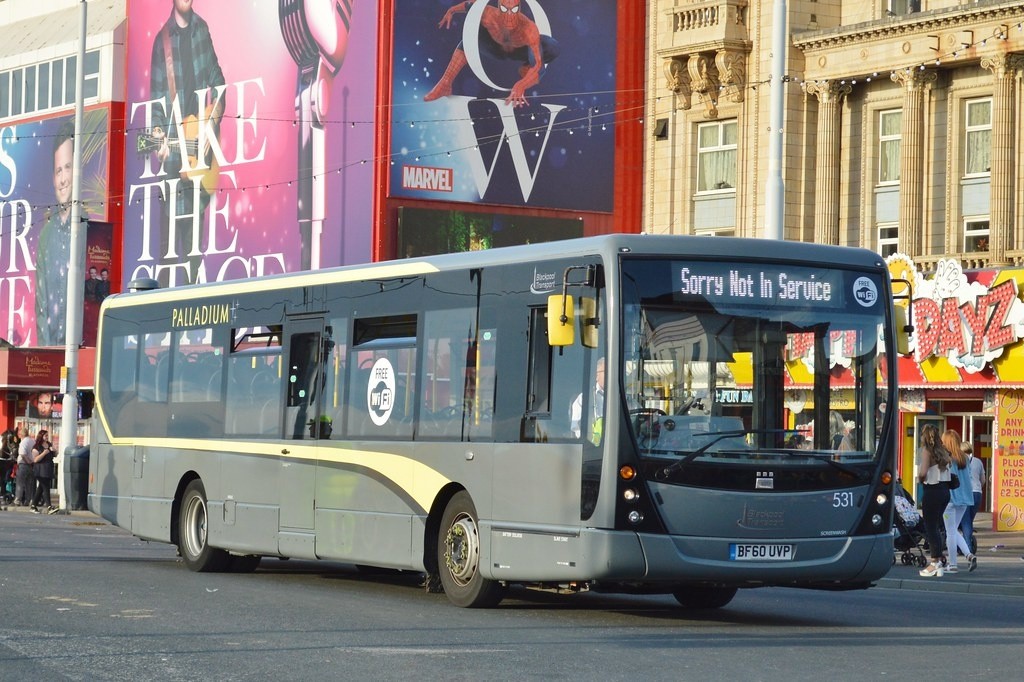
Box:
[87,232,914,609]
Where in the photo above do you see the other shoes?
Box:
[48,505,59,514]
[29,505,40,514]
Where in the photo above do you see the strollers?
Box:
[890,477,927,568]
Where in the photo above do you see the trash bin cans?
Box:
[64,444,90,510]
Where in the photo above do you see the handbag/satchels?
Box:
[946,473,960,490]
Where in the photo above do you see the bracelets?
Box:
[151,129,161,134]
[208,115,218,130]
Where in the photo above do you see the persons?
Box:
[29,429,60,515]
[84,266,110,302]
[918,423,952,577]
[28,391,63,418]
[941,429,978,573]
[424,0,560,107]
[830,410,851,460]
[147,0,226,288]
[12,427,35,506]
[960,441,985,554]
[571,357,642,441]
[34,122,89,346]
[784,434,807,449]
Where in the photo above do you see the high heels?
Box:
[919,560,944,577]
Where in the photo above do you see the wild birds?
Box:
[885,8,896,18]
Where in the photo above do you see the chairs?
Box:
[114,345,499,438]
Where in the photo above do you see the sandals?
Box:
[966,553,977,572]
[944,565,959,573]
